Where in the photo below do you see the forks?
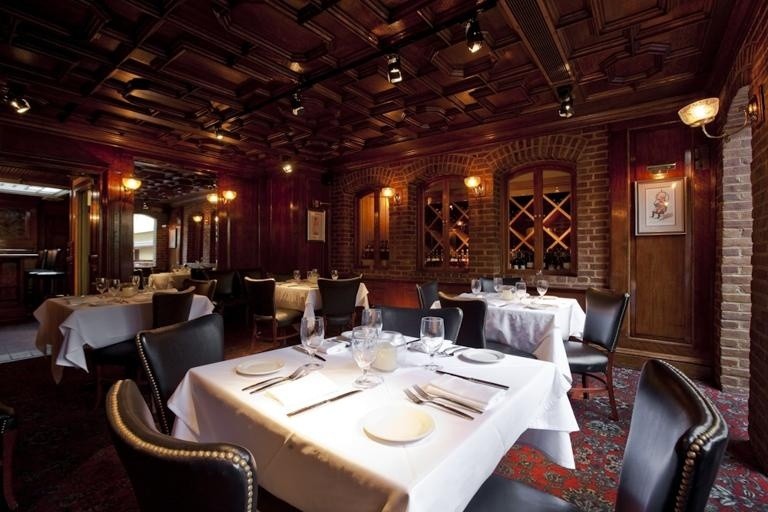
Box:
[446,348,466,357]
[242,364,309,391]
[249,365,324,394]
[439,345,458,355]
[405,388,474,420]
[414,384,483,414]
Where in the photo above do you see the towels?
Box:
[403,373,507,421]
[133,295,153,302]
[458,292,483,300]
[282,282,298,287]
[265,371,362,416]
[410,340,455,358]
[487,300,509,308]
[80,296,101,303]
[523,299,560,310]
[309,338,346,354]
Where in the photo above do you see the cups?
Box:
[106,279,121,297]
[129,275,140,287]
[312,268,317,272]
[331,270,338,280]
[306,271,312,282]
[95,277,108,294]
[420,317,444,370]
[294,270,301,282]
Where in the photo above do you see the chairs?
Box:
[136,314,225,437]
[23,249,68,300]
[317,277,362,331]
[152,285,197,328]
[416,279,439,310]
[183,278,217,302]
[245,277,302,351]
[204,270,245,305]
[563,289,631,420]
[464,357,729,511]
[235,268,264,295]
[373,306,462,344]
[439,290,536,359]
[479,277,521,293]
[105,378,257,512]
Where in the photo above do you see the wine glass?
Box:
[515,281,527,301]
[471,279,481,298]
[362,309,382,334]
[300,317,325,371]
[494,277,503,297]
[351,326,383,388]
[537,280,549,300]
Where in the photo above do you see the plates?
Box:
[527,303,557,309]
[342,330,352,338]
[463,348,505,363]
[236,359,286,375]
[364,407,434,441]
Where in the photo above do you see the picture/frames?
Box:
[307,208,326,242]
[633,176,689,237]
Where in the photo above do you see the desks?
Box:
[0,248,38,305]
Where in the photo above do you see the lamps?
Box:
[207,193,217,209]
[123,176,142,194]
[647,163,676,180]
[387,54,403,84]
[465,18,484,56]
[382,187,403,205]
[557,86,575,118]
[464,175,486,197]
[290,91,305,117]
[679,96,759,140]
[222,189,237,207]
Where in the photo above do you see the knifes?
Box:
[293,346,327,362]
[288,390,361,418]
